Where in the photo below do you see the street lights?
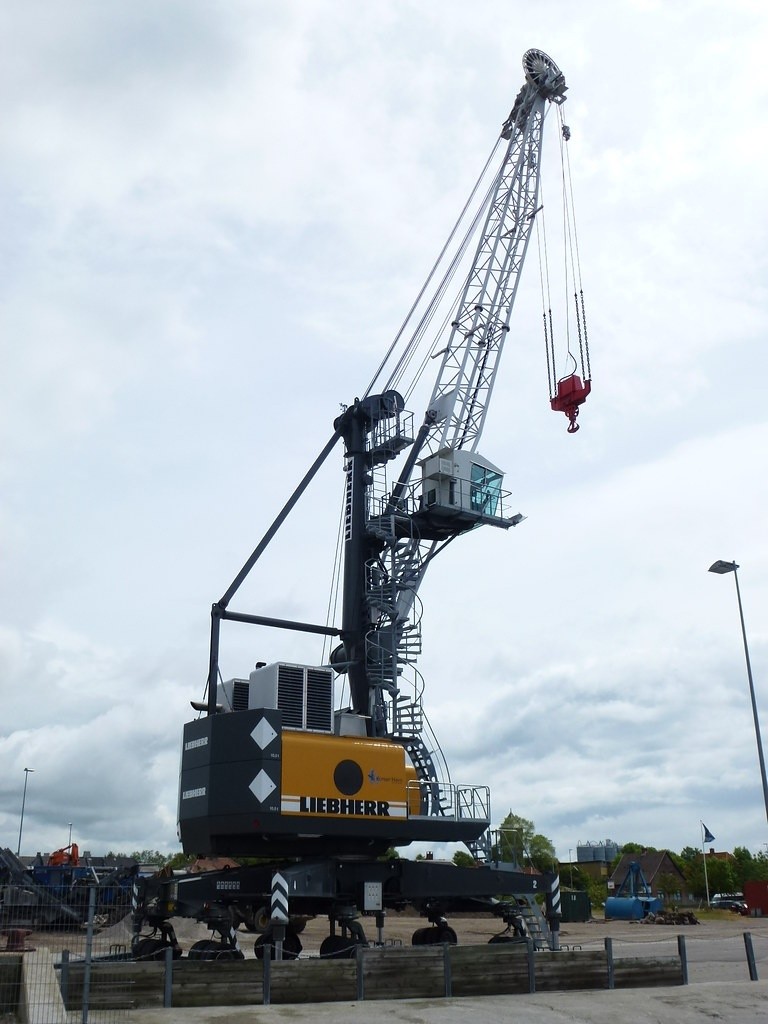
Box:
[706,559,768,818]
[67,822,73,856]
[16,766,35,859]
[569,849,573,891]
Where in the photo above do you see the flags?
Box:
[702,823,715,843]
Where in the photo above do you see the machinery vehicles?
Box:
[128,45,592,962]
